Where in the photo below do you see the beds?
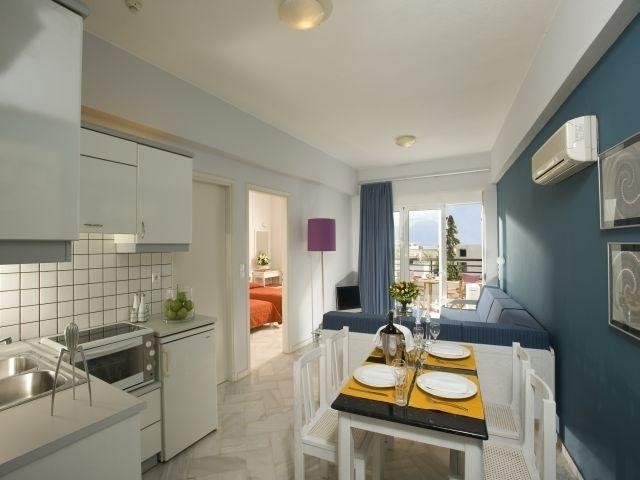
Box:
[250,282,282,330]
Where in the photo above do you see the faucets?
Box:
[0,336,13,345]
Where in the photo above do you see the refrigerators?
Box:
[155,323,219,462]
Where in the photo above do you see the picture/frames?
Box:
[597,132,640,229]
[607,242,640,340]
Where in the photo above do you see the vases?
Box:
[259,263,268,271]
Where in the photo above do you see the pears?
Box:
[164,292,193,320]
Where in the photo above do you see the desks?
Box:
[253,269,281,287]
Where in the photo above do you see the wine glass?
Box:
[391,322,441,406]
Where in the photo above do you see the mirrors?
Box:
[255,230,271,266]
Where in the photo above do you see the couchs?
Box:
[324,285,550,349]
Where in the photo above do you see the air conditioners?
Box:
[532,115,599,185]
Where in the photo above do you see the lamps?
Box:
[277,0,334,32]
[395,136,416,148]
[307,219,335,342]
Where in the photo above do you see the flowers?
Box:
[256,250,271,265]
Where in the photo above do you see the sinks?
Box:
[0,350,49,380]
[0,367,90,413]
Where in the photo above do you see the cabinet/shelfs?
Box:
[0,0,83,240]
[137,387,162,474]
[80,128,192,253]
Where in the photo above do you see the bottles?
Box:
[384,311,397,333]
[130,291,150,322]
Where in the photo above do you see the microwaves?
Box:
[41,319,156,394]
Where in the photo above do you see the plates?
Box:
[353,364,406,388]
[416,371,478,398]
[377,342,415,354]
[424,340,471,359]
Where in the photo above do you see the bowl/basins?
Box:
[163,287,196,324]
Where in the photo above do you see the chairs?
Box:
[450,341,556,480]
[293,325,394,480]
